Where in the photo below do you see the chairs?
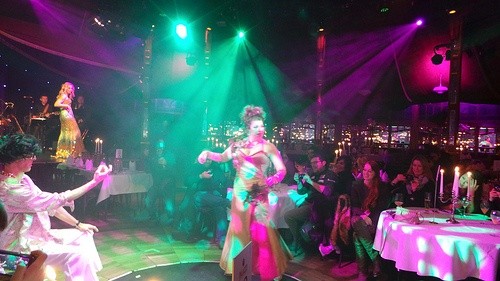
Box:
[50,167,95,215]
[311,193,380,281]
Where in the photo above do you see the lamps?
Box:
[433,73,448,94]
[431,42,453,65]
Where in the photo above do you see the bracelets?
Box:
[72,220,81,229]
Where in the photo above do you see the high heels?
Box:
[372,259,382,278]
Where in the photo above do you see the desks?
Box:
[226,184,308,228]
[373,206,500,281]
[52,164,153,219]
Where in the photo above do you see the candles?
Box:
[335,138,351,159]
[207,137,226,153]
[97,138,100,153]
[100,140,103,153]
[453,166,460,191]
[95,140,98,153]
[440,169,444,193]
[467,172,472,197]
[267,136,278,148]
[455,172,459,196]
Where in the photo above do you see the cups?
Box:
[115,149,123,159]
[395,193,403,206]
[424,193,431,209]
[129,161,136,171]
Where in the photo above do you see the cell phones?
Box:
[0,250,36,277]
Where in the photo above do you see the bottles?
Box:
[114,159,123,170]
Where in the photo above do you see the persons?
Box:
[73,96,89,131]
[178,146,229,246]
[0,139,112,281]
[198,106,290,281]
[283,138,388,281]
[387,145,500,214]
[31,96,51,151]
[54,81,85,159]
[0,250,48,281]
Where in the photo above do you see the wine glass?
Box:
[490,210,500,236]
[461,197,470,216]
[480,202,490,224]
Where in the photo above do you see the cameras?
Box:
[299,174,306,179]
[403,174,414,181]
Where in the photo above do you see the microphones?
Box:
[5,102,14,106]
